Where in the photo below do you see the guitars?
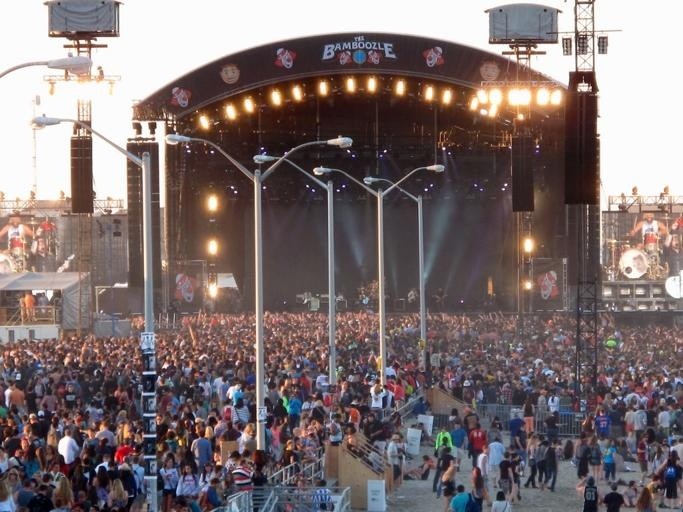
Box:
[362,293,372,304]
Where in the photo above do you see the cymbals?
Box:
[26,221,39,224]
[658,216,673,221]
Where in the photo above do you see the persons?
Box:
[0,214,39,256]
[26,239,56,272]
[658,234,682,276]
[0,277,682,512]
[624,212,680,250]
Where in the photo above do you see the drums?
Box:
[619,249,649,279]
[645,233,656,245]
[665,275,682,298]
[0,254,14,274]
[10,238,28,256]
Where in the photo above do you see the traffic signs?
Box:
[253,155,336,385]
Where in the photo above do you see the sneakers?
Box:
[524,481,556,493]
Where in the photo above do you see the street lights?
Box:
[313,164,445,384]
[0,55,93,79]
[164,133,353,451]
[30,115,158,512]
